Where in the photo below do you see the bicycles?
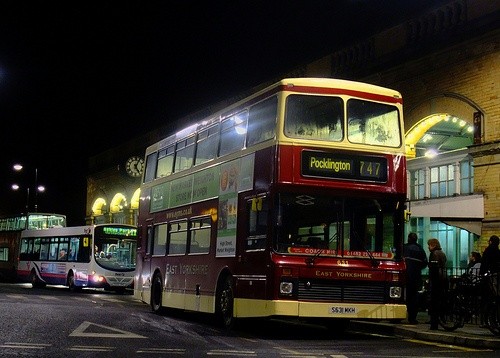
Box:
[438,272,500,336]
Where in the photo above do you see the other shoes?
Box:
[425,321,432,324]
[409,320,419,324]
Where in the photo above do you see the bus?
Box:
[133,78,412,331]
[0,213,66,282]
[18,223,137,292]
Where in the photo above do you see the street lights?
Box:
[14,164,45,213]
[12,184,29,213]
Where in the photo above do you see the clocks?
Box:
[118,152,144,178]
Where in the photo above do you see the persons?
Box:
[479,235,500,329]
[426,238,450,330]
[59,243,115,262]
[388,233,428,325]
[449,252,484,328]
